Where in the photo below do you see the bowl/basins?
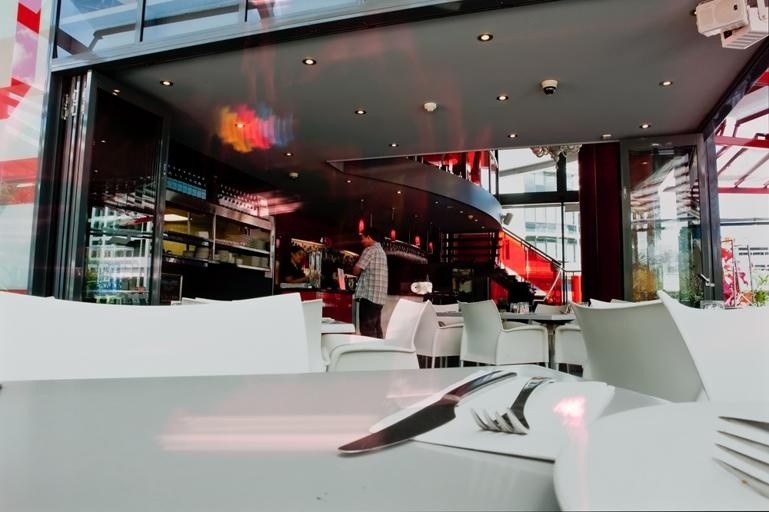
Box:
[214,250,268,269]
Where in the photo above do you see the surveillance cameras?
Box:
[721,0,769,49]
[541,79,559,95]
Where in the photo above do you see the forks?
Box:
[470,376,550,434]
[713,415,769,499]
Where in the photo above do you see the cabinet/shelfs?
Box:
[163,190,276,287]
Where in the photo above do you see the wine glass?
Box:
[381,237,428,265]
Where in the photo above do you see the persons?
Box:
[279,246,320,283]
[352,226,388,339]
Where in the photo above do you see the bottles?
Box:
[165,165,270,222]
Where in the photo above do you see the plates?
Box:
[552,400,769,512]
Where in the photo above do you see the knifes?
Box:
[338,370,518,452]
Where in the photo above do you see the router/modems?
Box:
[696,0,749,37]
